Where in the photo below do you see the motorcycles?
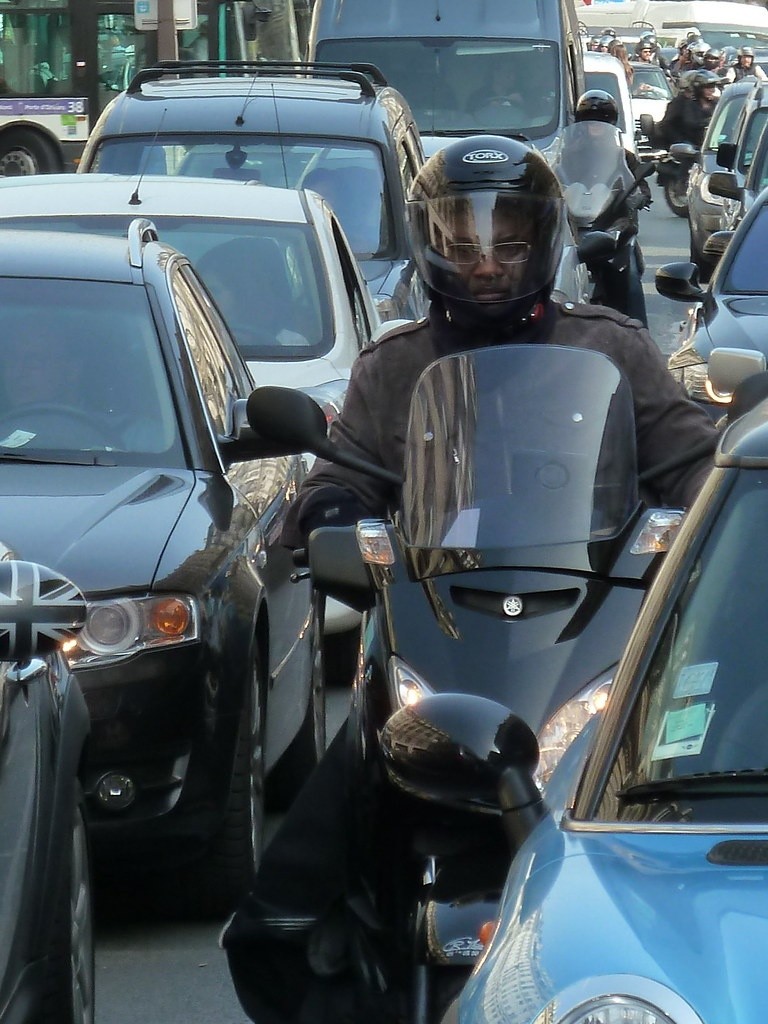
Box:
[545,121,656,312]
[657,142,698,217]
[246,343,689,1024]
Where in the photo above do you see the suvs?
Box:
[72,59,428,328]
[0,219,328,915]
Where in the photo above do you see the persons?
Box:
[578,21,768,187]
[227,133,723,1024]
[552,89,650,274]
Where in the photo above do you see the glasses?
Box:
[444,241,539,266]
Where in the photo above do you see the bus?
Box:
[0,0,301,177]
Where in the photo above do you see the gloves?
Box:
[300,499,367,543]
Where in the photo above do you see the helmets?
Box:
[404,134,564,300]
[575,90,618,126]
[592,28,754,103]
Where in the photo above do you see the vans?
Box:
[307,0,585,172]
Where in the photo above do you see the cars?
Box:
[656,187,768,423]
[580,50,654,162]
[0,560,97,1024]
[0,174,414,639]
[383,398,768,1024]
[670,80,767,285]
[626,61,675,164]
